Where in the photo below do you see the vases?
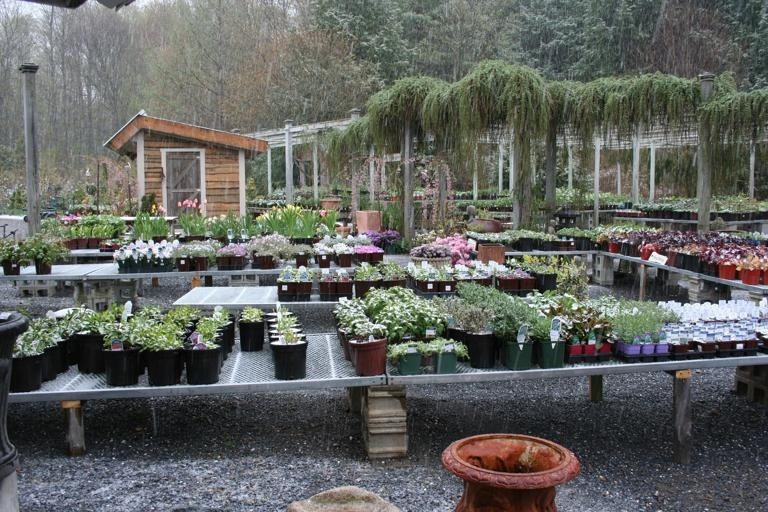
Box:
[276,272,557,301]
[296,255,381,267]
[216,256,246,270]
[256,256,274,268]
[179,257,208,271]
[118,260,174,273]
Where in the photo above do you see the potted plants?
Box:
[482,230,556,252]
[559,188,766,284]
[384,180,559,230]
[0,213,126,276]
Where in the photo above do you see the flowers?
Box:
[134,199,204,241]
[114,240,179,263]
[206,204,337,238]
[297,228,402,254]
[222,232,289,255]
[413,234,475,266]
[276,255,553,282]
[182,240,221,258]
